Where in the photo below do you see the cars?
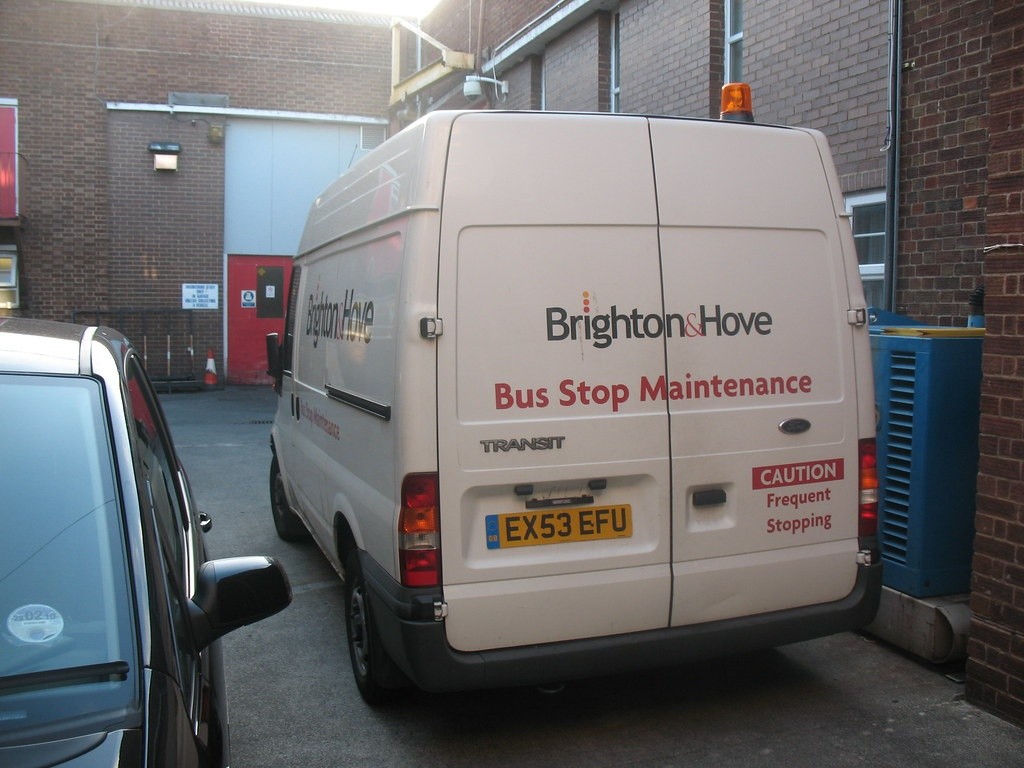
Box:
[2,318,293,766]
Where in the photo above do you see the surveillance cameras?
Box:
[463,81,482,102]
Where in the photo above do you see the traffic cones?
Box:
[200,348,225,393]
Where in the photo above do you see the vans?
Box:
[263,107,883,708]
